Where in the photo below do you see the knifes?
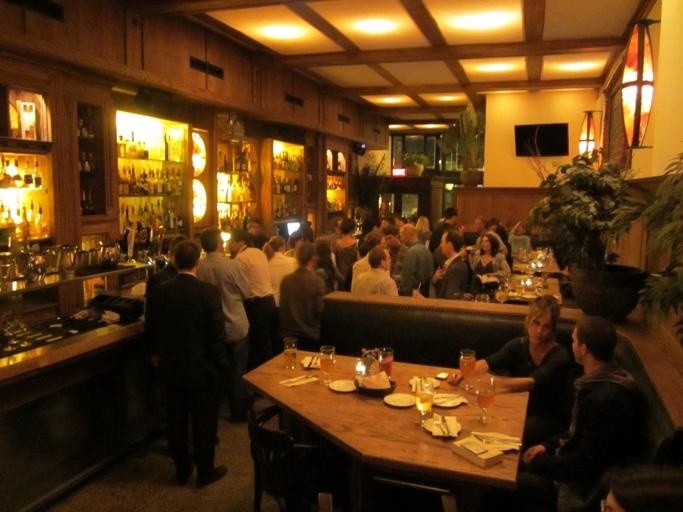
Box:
[436,415,450,436]
[307,355,316,369]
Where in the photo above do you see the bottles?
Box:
[273,152,304,219]
[308,157,312,185]
[78,109,182,235]
[218,150,250,172]
[223,179,256,202]
[0,151,46,239]
[219,205,251,232]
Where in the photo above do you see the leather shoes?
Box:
[214,465,227,480]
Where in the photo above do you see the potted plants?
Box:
[530,150,641,320]
[434,110,483,186]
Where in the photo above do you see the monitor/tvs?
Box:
[274,219,302,239]
[515,123,568,156]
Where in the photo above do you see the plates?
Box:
[478,431,514,454]
[422,421,462,434]
[431,393,461,408]
[328,378,357,393]
[383,392,416,409]
[410,376,441,391]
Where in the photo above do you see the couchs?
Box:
[324,301,682,512]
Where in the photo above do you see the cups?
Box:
[376,346,393,378]
[283,335,297,370]
[319,344,334,386]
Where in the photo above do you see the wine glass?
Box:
[473,247,545,305]
[415,387,433,430]
[469,373,497,429]
[458,348,476,386]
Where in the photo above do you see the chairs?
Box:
[352,468,456,510]
[247,413,335,512]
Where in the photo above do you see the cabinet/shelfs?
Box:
[216,140,307,231]
[0,84,189,247]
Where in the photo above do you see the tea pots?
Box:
[56,244,78,278]
[72,240,105,268]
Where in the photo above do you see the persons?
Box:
[142,207,513,488]
[448,207,682,512]
